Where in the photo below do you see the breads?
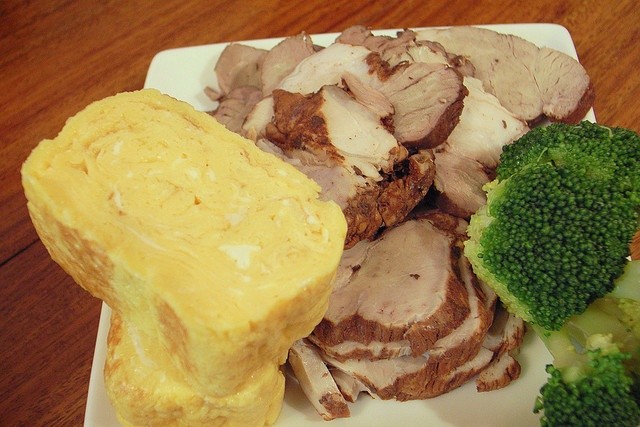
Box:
[103,305,286,427]
[19,87,348,398]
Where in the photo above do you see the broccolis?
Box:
[464,118,639,336]
[530,257,640,426]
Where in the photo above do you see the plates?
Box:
[84,24,631,427]
